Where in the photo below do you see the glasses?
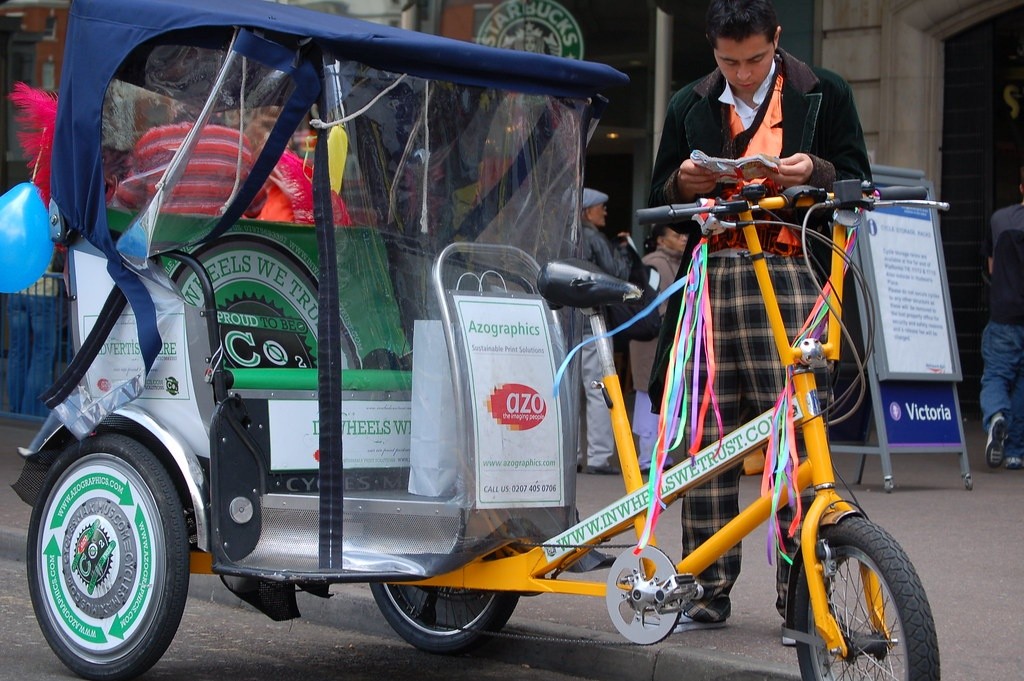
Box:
[598,205,607,211]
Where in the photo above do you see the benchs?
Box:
[105,207,414,392]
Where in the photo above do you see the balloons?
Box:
[0,182,54,294]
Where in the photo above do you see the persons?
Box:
[241,115,353,226]
[645,1,874,648]
[561,185,640,475]
[979,170,1024,468]
[7,274,63,417]
[629,221,688,472]
[107,95,265,220]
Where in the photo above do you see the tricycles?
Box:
[8,3,954,681]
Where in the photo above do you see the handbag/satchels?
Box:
[609,234,662,341]
[407,269,508,498]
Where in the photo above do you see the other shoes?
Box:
[586,462,620,475]
[783,624,797,643]
[635,607,727,633]
[577,464,582,473]
[641,465,674,475]
[1006,457,1024,468]
[985,415,1008,468]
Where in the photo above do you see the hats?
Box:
[560,187,608,211]
